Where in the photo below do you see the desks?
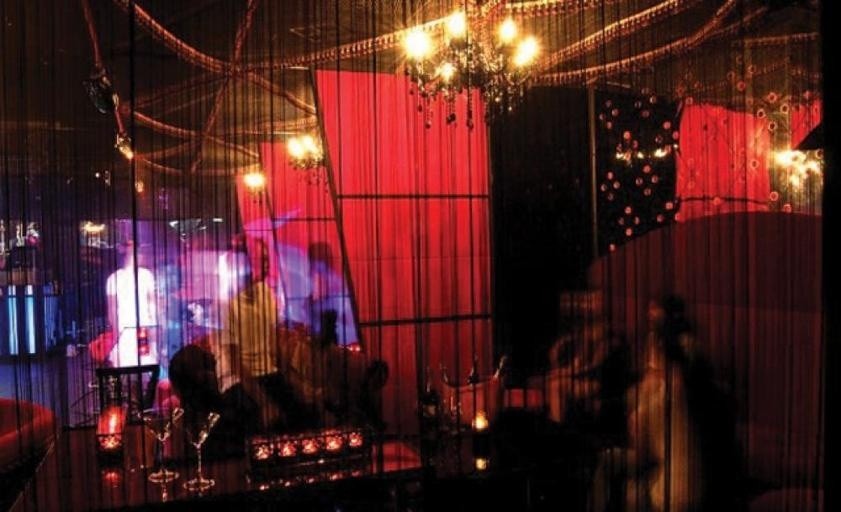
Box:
[95,324,162,415]
[10,425,425,512]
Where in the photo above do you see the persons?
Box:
[105,241,159,396]
[626,290,714,512]
[169,241,281,467]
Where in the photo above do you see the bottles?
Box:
[423,354,510,418]
[138,327,149,356]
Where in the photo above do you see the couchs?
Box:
[565,210,826,510]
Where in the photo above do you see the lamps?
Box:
[403,0,539,130]
[287,126,324,169]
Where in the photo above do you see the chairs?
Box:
[0,396,56,479]
[192,319,389,425]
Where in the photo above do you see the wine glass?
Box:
[138,407,220,491]
